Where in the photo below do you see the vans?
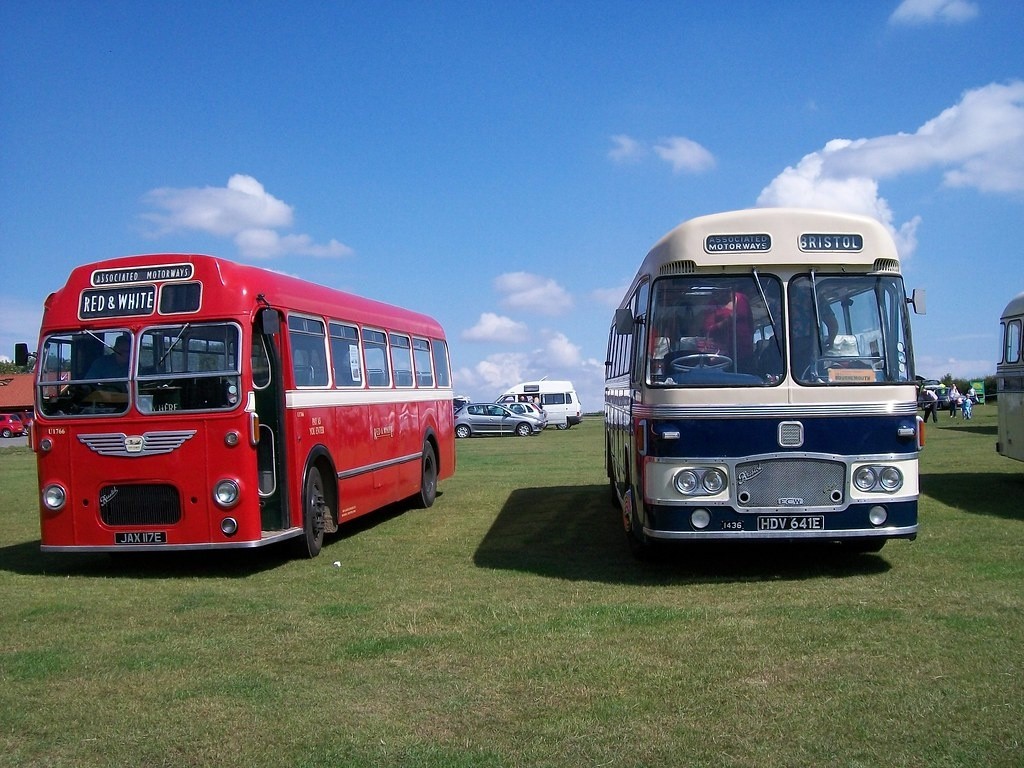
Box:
[491,376,583,430]
[453,395,472,416]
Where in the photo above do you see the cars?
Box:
[15,412,34,435]
[0,413,25,438]
[476,402,548,433]
[455,402,544,438]
[957,392,976,407]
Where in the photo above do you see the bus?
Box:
[994,293,1024,466]
[920,379,944,411]
[600,207,930,553]
[14,251,458,560]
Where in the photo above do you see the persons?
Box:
[915,384,920,403]
[767,279,840,380]
[83,336,131,396]
[704,279,755,374]
[920,388,939,424]
[968,385,979,405]
[533,397,542,408]
[960,394,972,419]
[947,384,960,418]
